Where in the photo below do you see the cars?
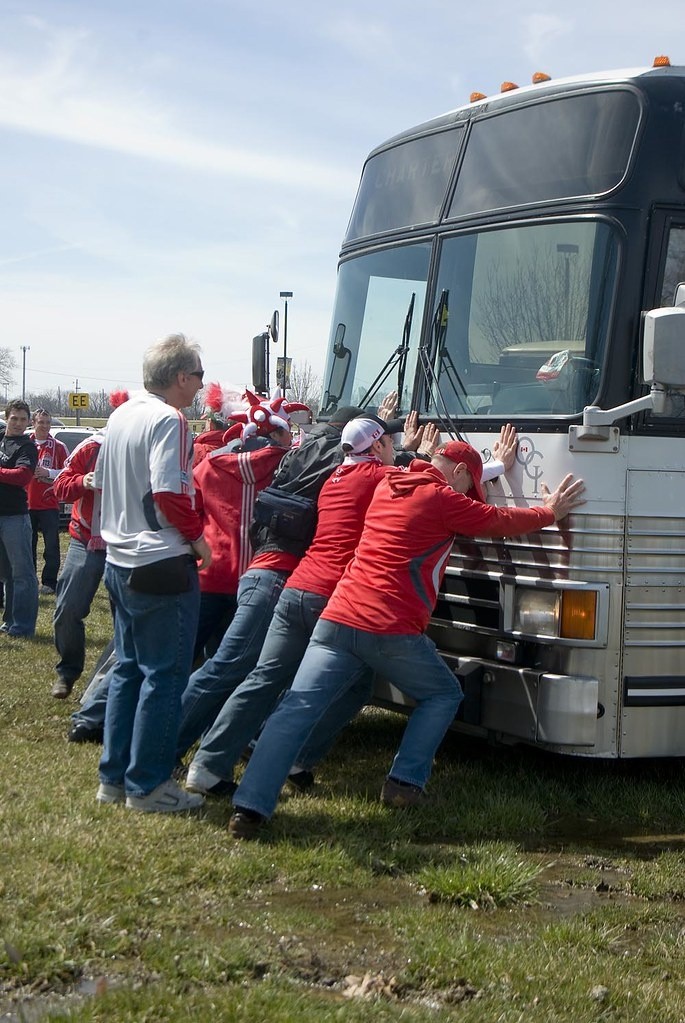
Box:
[0,410,100,528]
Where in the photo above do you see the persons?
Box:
[93,337,213,816]
[0,395,587,837]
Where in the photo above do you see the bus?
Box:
[315,54,685,761]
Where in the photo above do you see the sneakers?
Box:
[95,781,123,803]
[125,775,205,812]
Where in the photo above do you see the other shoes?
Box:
[5,632,25,638]
[286,770,314,792]
[173,759,188,778]
[40,585,54,594]
[185,776,239,798]
[0,622,11,632]
[228,807,273,839]
[380,776,434,804]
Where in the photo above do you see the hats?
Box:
[434,441,487,504]
[201,383,250,426]
[222,389,310,445]
[328,405,366,423]
[340,413,402,453]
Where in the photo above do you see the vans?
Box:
[490,340,587,405]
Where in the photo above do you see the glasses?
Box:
[189,371,204,381]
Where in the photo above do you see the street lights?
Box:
[280,291,294,398]
[556,243,579,341]
[3,382,10,404]
[20,345,30,403]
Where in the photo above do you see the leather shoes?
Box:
[69,721,92,741]
[51,675,74,697]
